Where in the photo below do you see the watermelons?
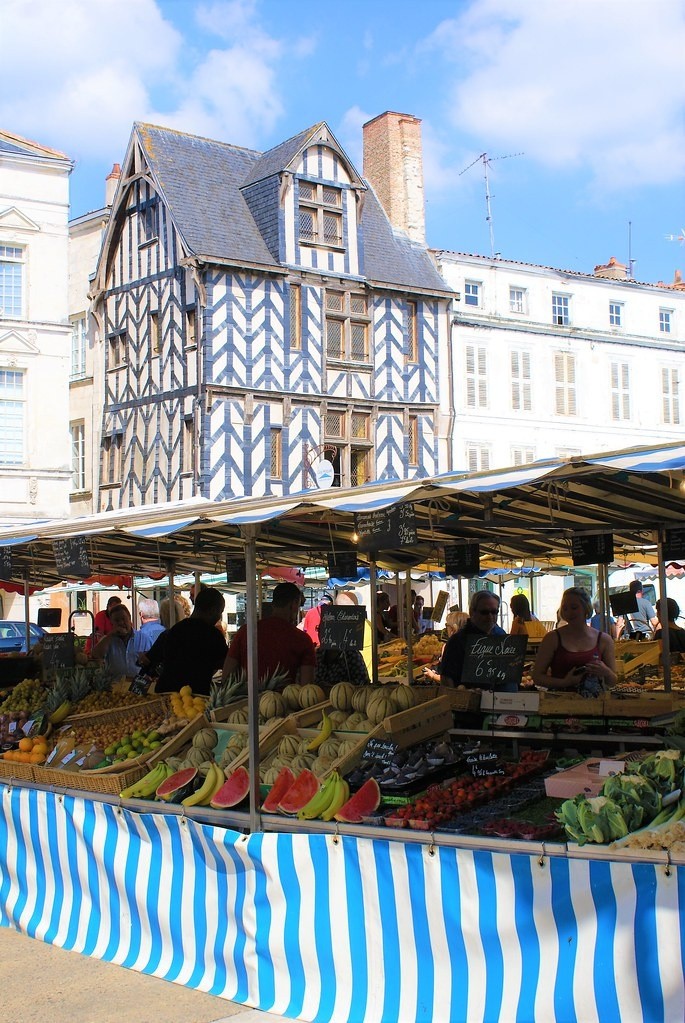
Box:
[334,777,381,823]
[155,766,198,803]
[210,765,320,818]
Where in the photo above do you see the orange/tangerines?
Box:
[2,736,48,765]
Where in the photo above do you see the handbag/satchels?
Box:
[579,674,605,697]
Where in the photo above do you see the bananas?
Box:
[182,758,225,808]
[118,760,175,804]
[297,768,349,821]
[306,707,333,751]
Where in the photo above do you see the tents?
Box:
[0,440,685,832]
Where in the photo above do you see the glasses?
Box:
[474,608,499,614]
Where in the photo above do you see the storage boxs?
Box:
[0,638,685,841]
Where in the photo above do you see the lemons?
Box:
[171,684,211,720]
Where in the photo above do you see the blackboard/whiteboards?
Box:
[444,542,481,576]
[227,556,247,584]
[129,675,152,697]
[457,633,530,686]
[319,604,368,651]
[609,591,640,617]
[662,527,685,560]
[52,534,92,577]
[362,738,399,764]
[41,631,76,669]
[466,752,499,763]
[571,534,616,565]
[326,552,358,577]
[0,546,13,583]
[353,501,417,552]
[471,760,506,777]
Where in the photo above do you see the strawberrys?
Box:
[382,748,547,829]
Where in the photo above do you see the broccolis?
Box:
[553,748,684,847]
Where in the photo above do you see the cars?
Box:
[0,619,49,654]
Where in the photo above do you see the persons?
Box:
[623,580,665,642]
[532,587,619,697]
[190,582,227,641]
[89,603,154,684]
[94,597,133,638]
[304,589,568,645]
[173,596,191,622]
[223,582,315,688]
[590,598,617,644]
[136,595,190,671]
[146,587,237,698]
[439,590,520,692]
[314,636,371,685]
[651,598,685,662]
